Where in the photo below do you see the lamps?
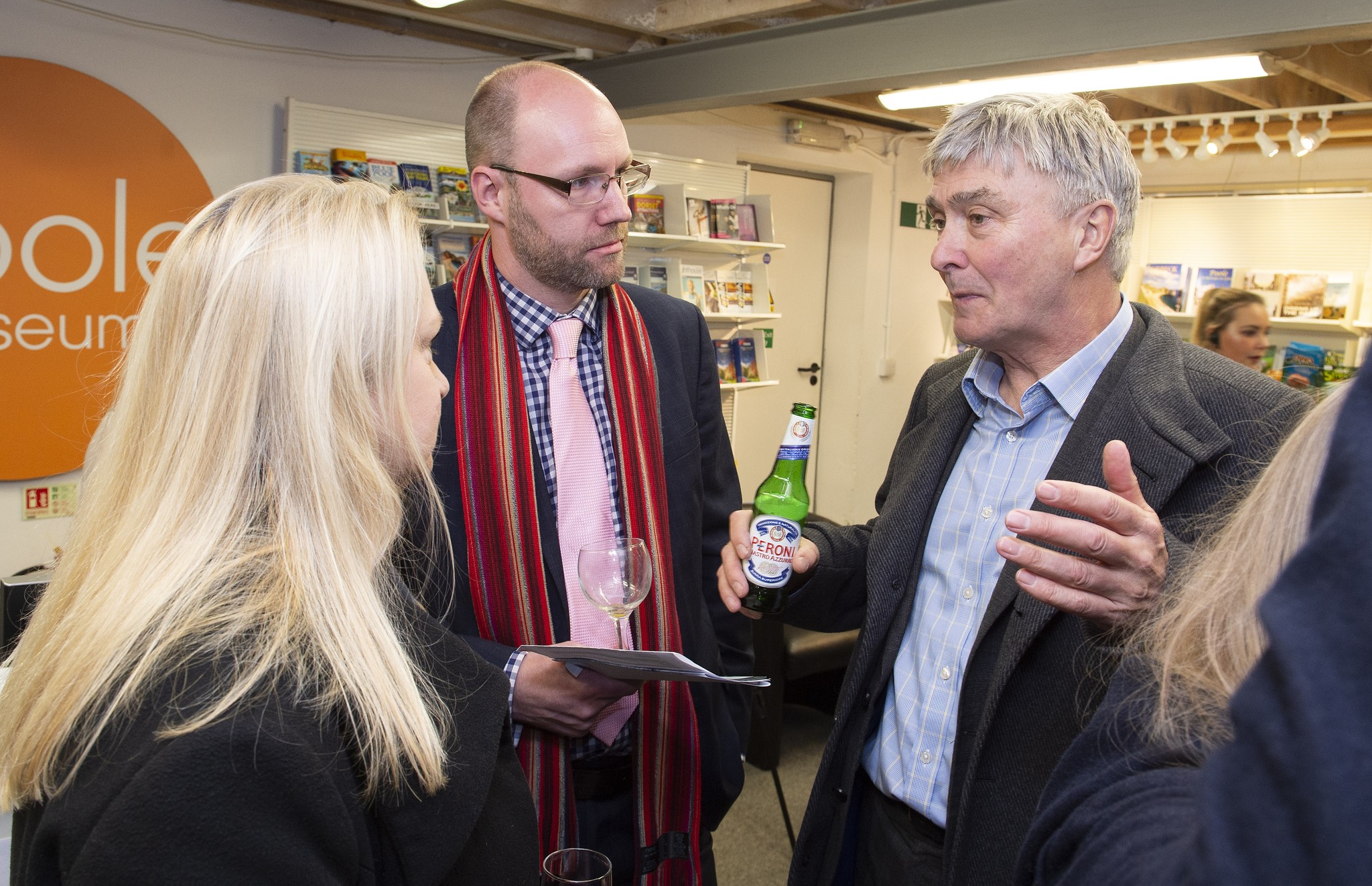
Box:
[1119,110,1333,156]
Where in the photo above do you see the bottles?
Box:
[740,402,816,613]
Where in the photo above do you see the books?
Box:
[625,198,759,242]
[1137,264,1356,320]
[1262,341,1356,404]
[712,337,759,384]
[421,232,483,288]
[296,148,476,222]
[622,264,774,312]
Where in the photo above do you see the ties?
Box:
[546,318,637,755]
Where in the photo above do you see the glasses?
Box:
[490,159,651,204]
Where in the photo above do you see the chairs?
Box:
[754,618,860,858]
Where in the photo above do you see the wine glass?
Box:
[578,537,654,649]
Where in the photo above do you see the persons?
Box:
[717,91,1315,886]
[1189,287,1270,369]
[0,174,539,886]
[1017,343,1372,886]
[392,62,771,886]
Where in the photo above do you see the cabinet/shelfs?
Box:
[280,95,786,450]
[1131,266,1372,387]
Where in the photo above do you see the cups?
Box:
[542,848,615,886]
